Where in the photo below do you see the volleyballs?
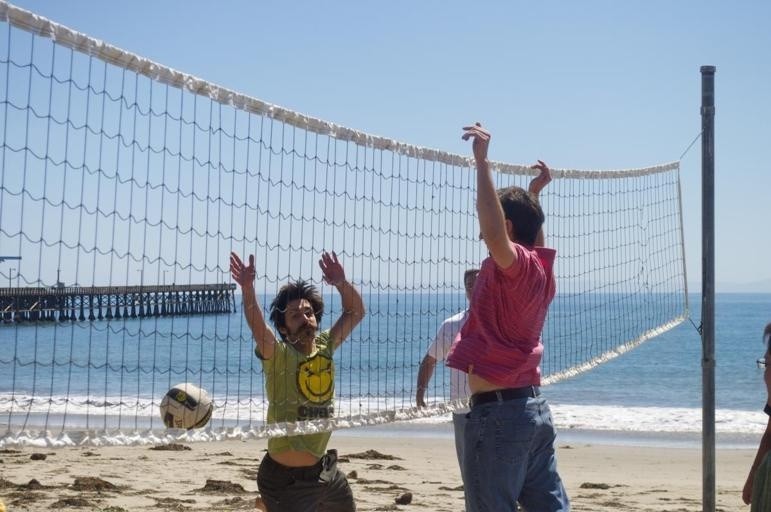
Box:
[160,383,212,431]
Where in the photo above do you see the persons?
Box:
[740,323,771,512]
[414,269,483,466]
[227,249,367,511]
[460,120,571,512]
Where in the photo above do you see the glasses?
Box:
[757,357,771,371]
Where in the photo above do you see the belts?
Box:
[265,452,336,476]
[467,385,541,409]
